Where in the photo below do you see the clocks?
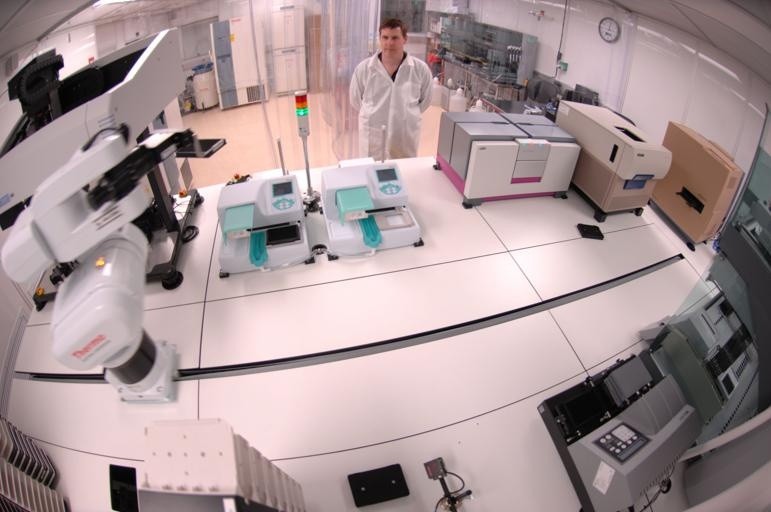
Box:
[599,18,621,44]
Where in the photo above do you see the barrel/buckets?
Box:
[431,77,484,113]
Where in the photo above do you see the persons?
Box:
[349,19,434,159]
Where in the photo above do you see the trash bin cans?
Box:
[192,63,218,110]
